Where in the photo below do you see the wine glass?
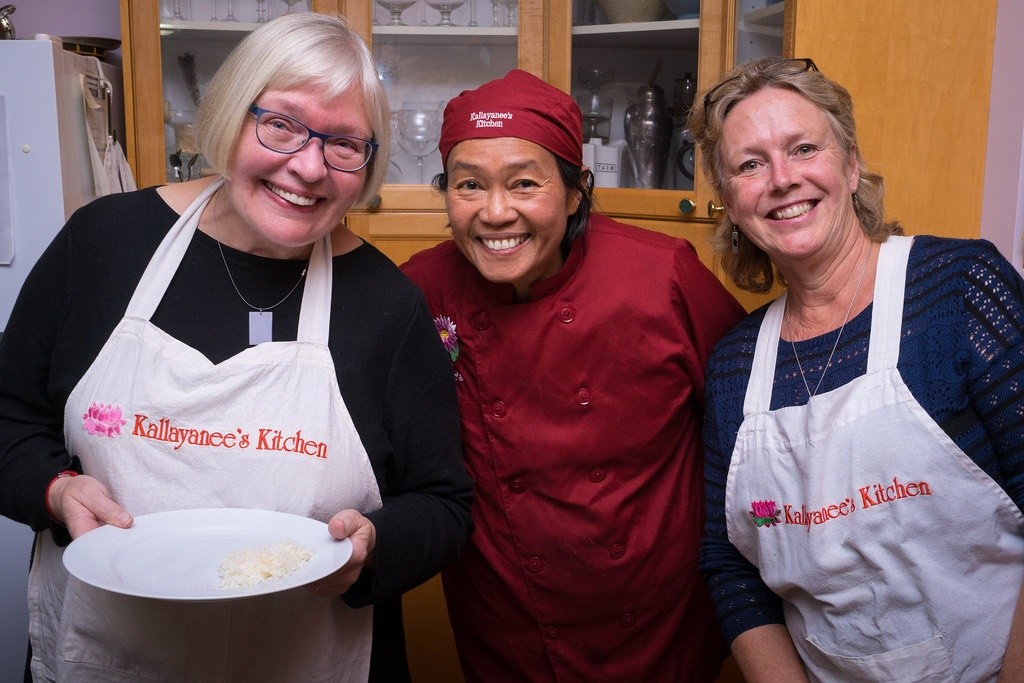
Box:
[391,98,442,183]
[574,61,615,145]
[162,1,517,29]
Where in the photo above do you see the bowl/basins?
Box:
[593,1,668,24]
[660,1,699,20]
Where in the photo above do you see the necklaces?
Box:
[213,184,309,309]
[771,238,874,397]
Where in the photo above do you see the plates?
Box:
[62,505,354,603]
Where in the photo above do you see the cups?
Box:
[581,138,628,190]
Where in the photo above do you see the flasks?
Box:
[624,84,673,190]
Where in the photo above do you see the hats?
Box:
[440,70,584,167]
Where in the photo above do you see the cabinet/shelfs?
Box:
[119,0,797,246]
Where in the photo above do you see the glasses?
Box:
[249,102,379,173]
[703,58,819,124]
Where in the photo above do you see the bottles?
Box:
[675,73,697,189]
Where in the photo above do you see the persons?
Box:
[689,57,1023,682]
[1,12,475,683]
[399,67,748,683]
